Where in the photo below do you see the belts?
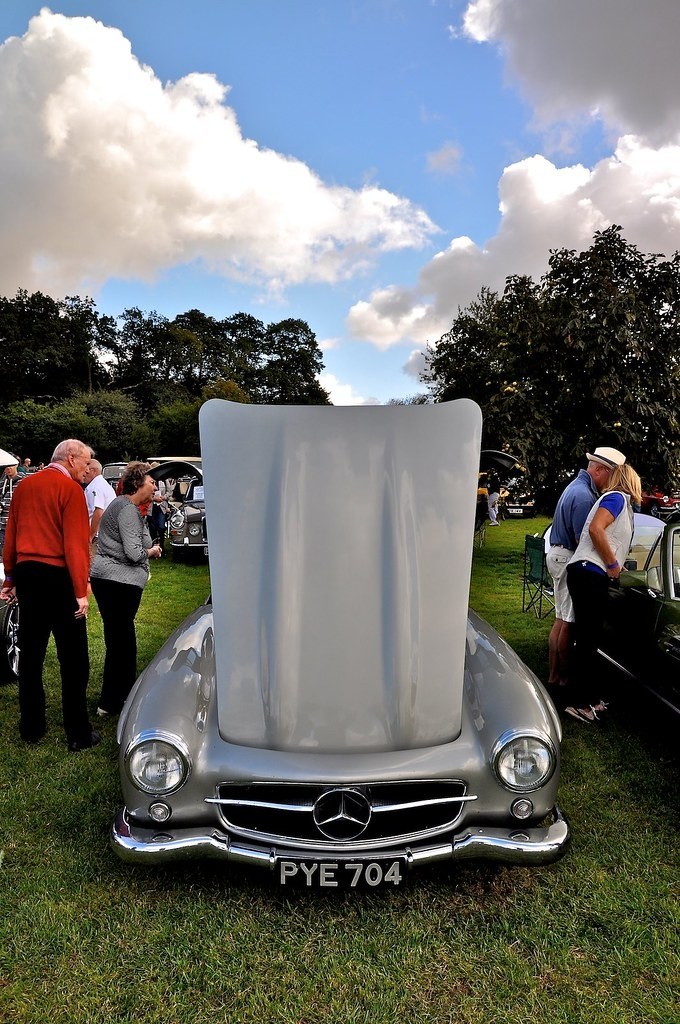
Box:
[550,543,574,551]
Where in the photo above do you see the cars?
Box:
[101,455,209,560]
[476,471,537,518]
[638,486,680,525]
[626,512,680,575]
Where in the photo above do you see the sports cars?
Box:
[109,397,569,897]
[590,516,680,735]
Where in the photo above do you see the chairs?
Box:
[522,535,555,620]
[473,501,489,550]
[648,566,680,598]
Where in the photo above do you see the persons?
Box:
[487,468,500,526]
[546,447,643,724]
[0,439,173,751]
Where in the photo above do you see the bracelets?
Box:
[151,548,155,556]
[606,560,619,569]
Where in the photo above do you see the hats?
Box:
[586,447,626,471]
[151,461,160,469]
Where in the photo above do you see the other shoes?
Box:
[501,515,505,521]
[548,671,569,686]
[488,522,499,526]
[565,702,607,724]
[68,732,101,751]
